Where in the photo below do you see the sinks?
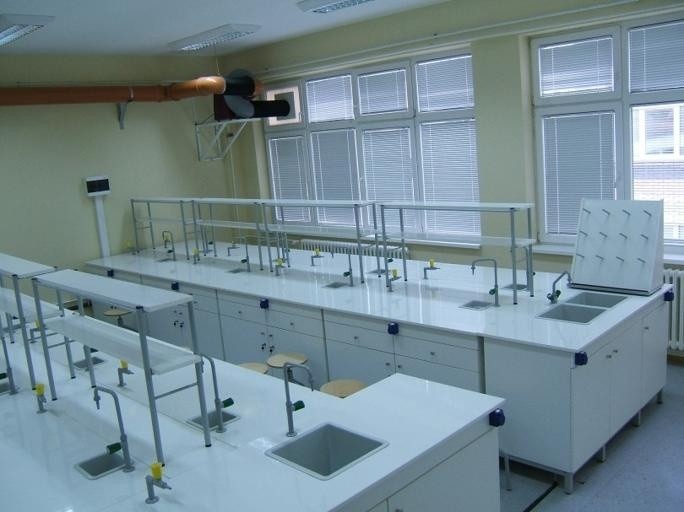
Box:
[565,292,631,307]
[536,304,606,324]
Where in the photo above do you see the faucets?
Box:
[150,226,538,298]
[2,298,326,511]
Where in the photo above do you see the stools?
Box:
[319,376,362,400]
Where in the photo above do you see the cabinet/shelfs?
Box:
[32,268,209,464]
[197,198,282,269]
[132,196,200,260]
[322,311,482,404]
[367,426,500,512]
[380,201,532,303]
[261,198,381,284]
[485,305,669,473]
[84,266,328,388]
[1,255,73,385]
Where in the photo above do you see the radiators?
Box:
[662,268,684,366]
